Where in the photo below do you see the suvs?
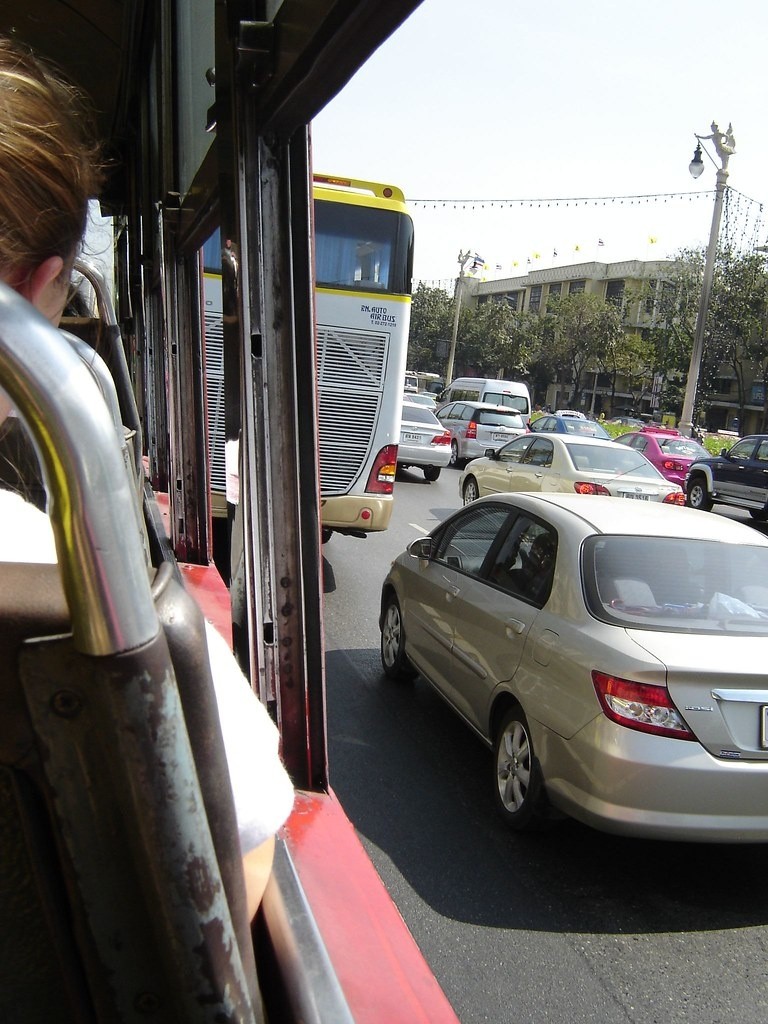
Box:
[686,433,768,520]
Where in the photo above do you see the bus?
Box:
[198,171,415,546]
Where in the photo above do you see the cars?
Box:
[459,433,685,511]
[378,492,768,845]
[397,402,453,481]
[612,428,712,494]
[531,410,613,443]
[402,393,436,414]
[435,401,530,467]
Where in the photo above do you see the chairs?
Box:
[573,456,590,468]
[568,426,574,432]
[494,533,556,599]
[619,461,635,472]
[0,262,262,1024]
[661,446,670,454]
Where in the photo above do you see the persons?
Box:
[598,408,605,420]
[0,35,296,926]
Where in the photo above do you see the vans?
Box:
[437,377,531,424]
[404,370,443,393]
